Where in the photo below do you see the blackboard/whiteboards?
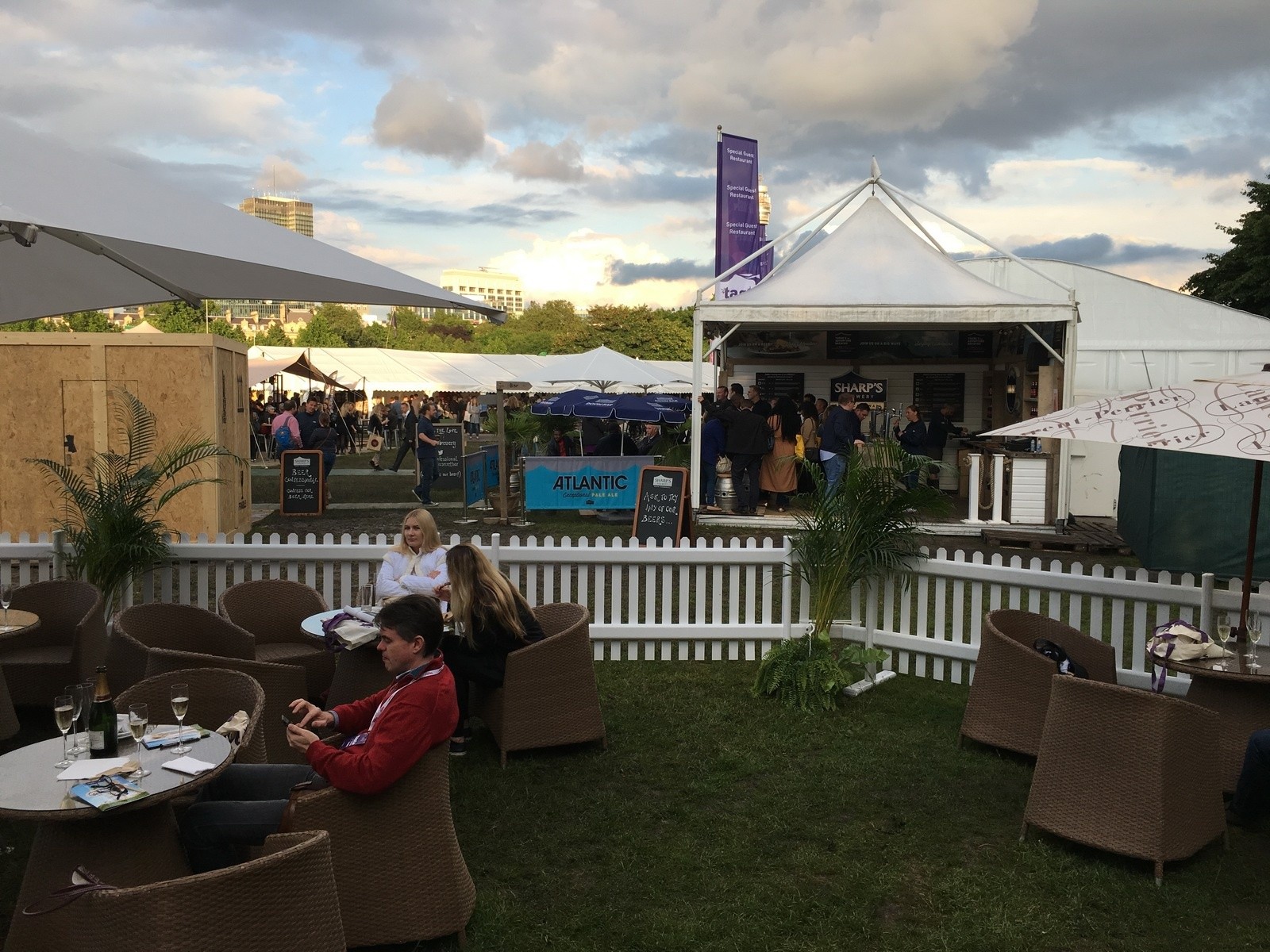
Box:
[281,450,325,516]
[912,373,966,422]
[632,466,691,549]
[415,422,464,491]
[756,372,804,406]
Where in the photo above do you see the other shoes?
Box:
[463,727,473,741]
[326,492,331,500]
[348,448,356,453]
[370,460,384,470]
[706,506,722,511]
[388,467,397,472]
[1224,800,1257,828]
[731,506,756,514]
[336,452,345,454]
[778,508,785,512]
[449,740,467,755]
[701,504,708,508]
[757,501,768,506]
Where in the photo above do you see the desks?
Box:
[300,606,453,738]
[0,724,235,952]
[1147,641,1270,793]
[0,609,41,741]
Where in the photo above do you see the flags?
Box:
[716,131,774,283]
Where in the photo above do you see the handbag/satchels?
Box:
[366,426,385,452]
[322,612,382,652]
[1147,619,1236,693]
[715,454,732,473]
[1032,637,1076,676]
[796,463,816,493]
[275,416,298,458]
[22,864,119,916]
[805,447,819,462]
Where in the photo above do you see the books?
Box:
[71,776,150,811]
[142,724,210,748]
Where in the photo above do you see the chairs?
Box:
[144,648,310,766]
[218,579,335,697]
[470,603,607,770]
[0,579,101,707]
[958,609,1116,756]
[107,603,256,700]
[1020,674,1229,886]
[71,831,347,951]
[114,668,268,814]
[294,738,476,948]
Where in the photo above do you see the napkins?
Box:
[56,757,130,780]
[161,755,217,775]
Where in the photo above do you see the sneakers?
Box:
[423,501,440,506]
[412,489,423,502]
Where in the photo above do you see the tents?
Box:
[691,156,1081,533]
[247,345,720,422]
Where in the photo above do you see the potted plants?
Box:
[481,408,540,515]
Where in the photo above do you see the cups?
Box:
[361,584,373,611]
[864,435,870,443]
[87,677,97,706]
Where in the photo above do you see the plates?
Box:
[118,714,133,739]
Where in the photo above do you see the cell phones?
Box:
[281,714,297,734]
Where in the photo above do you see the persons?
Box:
[249,391,547,506]
[182,594,458,874]
[699,383,927,516]
[927,401,968,480]
[89,784,143,796]
[432,543,546,756]
[544,417,667,456]
[376,508,450,612]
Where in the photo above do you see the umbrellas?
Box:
[625,359,707,395]
[563,392,688,456]
[530,388,608,456]
[0,116,508,329]
[975,362,1270,639]
[542,345,682,394]
[647,393,690,414]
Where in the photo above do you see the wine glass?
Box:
[0,584,13,630]
[65,685,87,755]
[1246,617,1262,667]
[78,683,92,752]
[171,683,192,754]
[1217,615,1231,666]
[1243,610,1259,658]
[128,703,150,778]
[54,695,76,768]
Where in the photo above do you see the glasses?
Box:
[92,775,128,800]
[851,400,856,404]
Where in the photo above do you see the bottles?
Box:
[1031,407,1038,418]
[1037,438,1041,452]
[1030,381,1038,398]
[1031,438,1035,452]
[89,665,117,759]
